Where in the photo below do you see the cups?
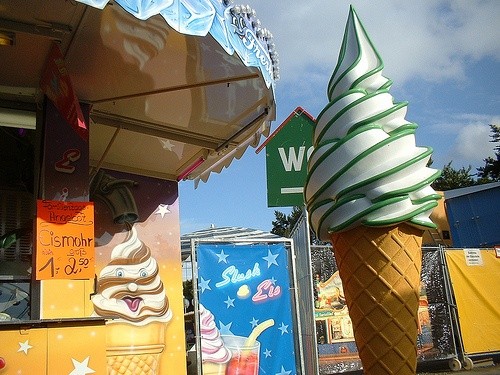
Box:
[221,335,260,375]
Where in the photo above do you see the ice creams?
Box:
[199,303,232,375]
[303,5,443,375]
[91,220,173,375]
